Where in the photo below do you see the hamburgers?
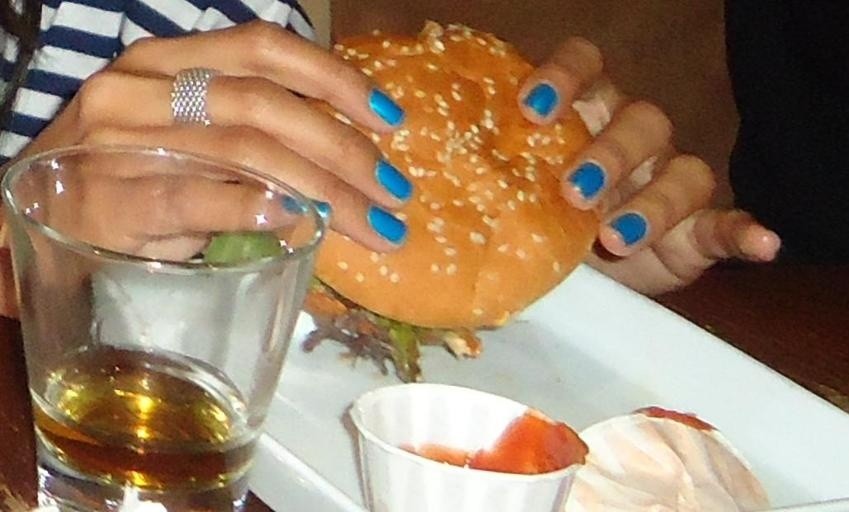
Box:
[264,16,610,382]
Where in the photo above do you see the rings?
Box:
[170,67,223,128]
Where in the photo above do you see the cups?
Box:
[350,382,592,510]
[0,143,325,512]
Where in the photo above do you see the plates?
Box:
[88,259,847,509]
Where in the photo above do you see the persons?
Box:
[0,1,785,325]
